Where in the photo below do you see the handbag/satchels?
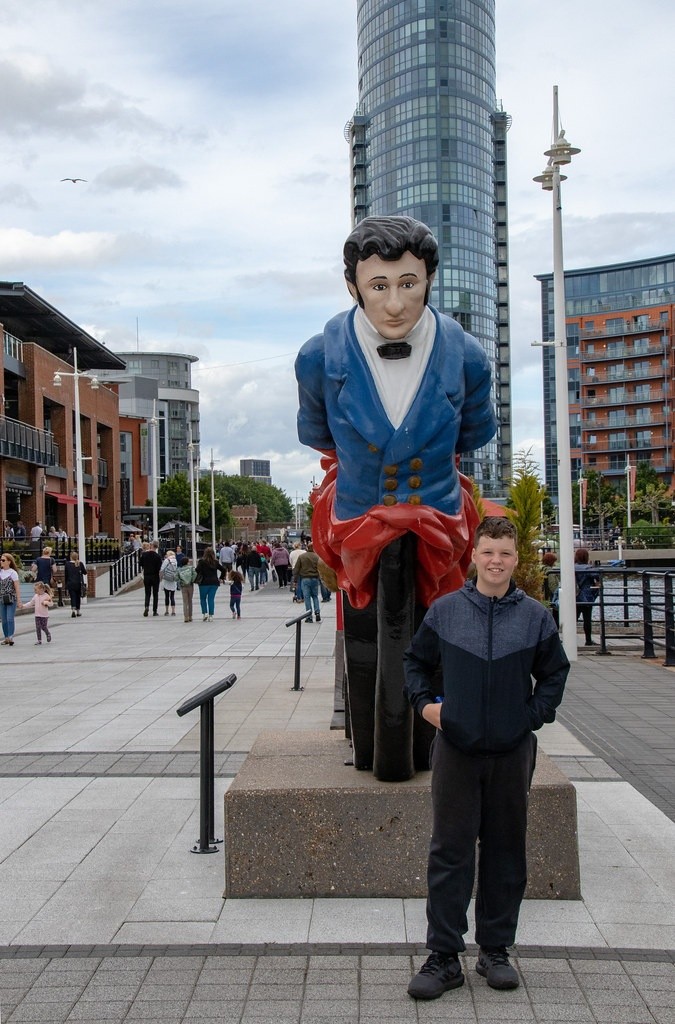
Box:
[194,575,204,585]
[80,584,87,598]
[2,594,15,607]
[271,570,277,582]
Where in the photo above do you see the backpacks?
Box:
[162,560,177,582]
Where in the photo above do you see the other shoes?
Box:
[203,613,209,622]
[184,617,189,623]
[232,612,237,620]
[207,615,214,622]
[237,616,241,620]
[171,613,176,616]
[1,638,9,645]
[189,618,193,621]
[77,614,83,617]
[315,612,321,621]
[35,641,42,646]
[9,638,15,647]
[164,612,169,616]
[144,608,149,617]
[152,613,159,616]
[71,612,76,618]
[47,633,52,643]
[305,618,313,623]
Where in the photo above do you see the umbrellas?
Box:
[158,519,212,534]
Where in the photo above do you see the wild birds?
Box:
[61,178,88,184]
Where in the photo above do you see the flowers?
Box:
[18,568,38,583]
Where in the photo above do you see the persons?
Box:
[31,548,57,594]
[403,517,572,999]
[65,552,87,618]
[4,520,79,547]
[123,533,332,622]
[294,215,499,785]
[541,547,600,646]
[591,525,621,551]
[22,581,53,645]
[0,553,22,646]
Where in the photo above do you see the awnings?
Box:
[121,522,142,532]
[46,491,100,506]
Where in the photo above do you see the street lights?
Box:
[530,83,582,665]
[209,447,216,557]
[576,469,584,539]
[50,346,100,604]
[149,398,158,556]
[624,455,632,527]
[186,430,198,569]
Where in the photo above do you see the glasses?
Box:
[0,559,9,563]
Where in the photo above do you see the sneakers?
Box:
[407,951,466,1001]
[475,946,520,990]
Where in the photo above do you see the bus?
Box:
[283,529,307,550]
